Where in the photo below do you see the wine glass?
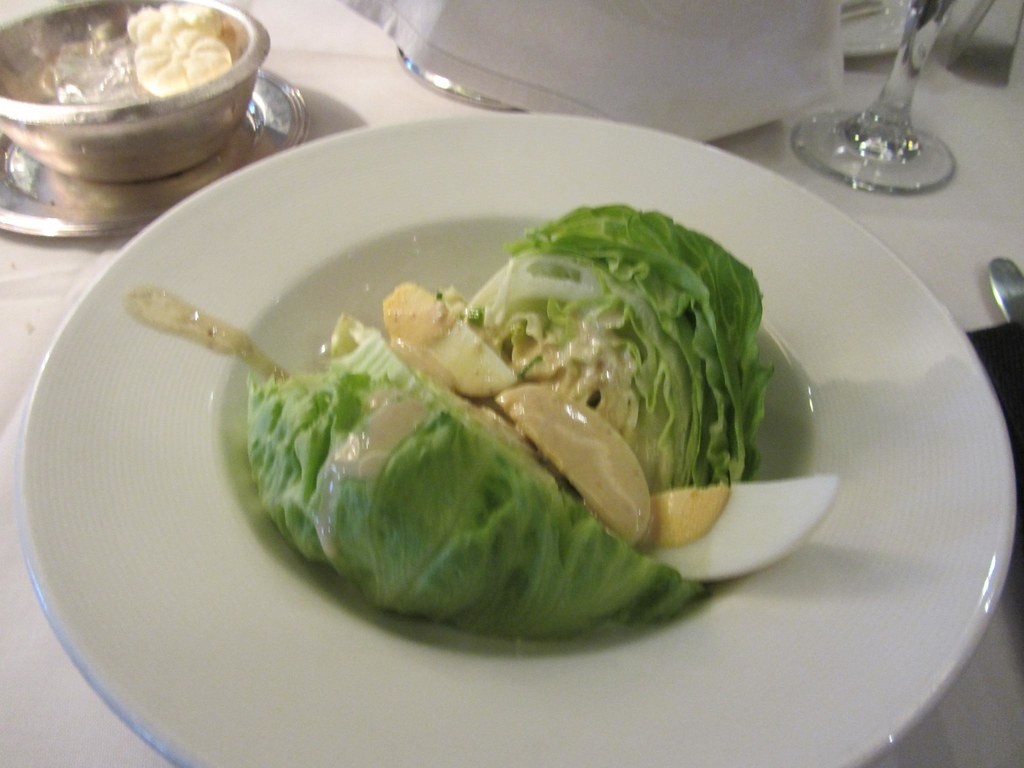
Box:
[790,1,956,197]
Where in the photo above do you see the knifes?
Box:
[987,258,1024,336]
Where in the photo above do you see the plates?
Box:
[16,113,1013,768]
[0,70,310,238]
[839,2,910,58]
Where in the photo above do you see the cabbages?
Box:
[246,205,772,641]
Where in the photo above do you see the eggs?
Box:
[609,471,842,582]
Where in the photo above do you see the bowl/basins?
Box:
[0,1,271,185]
